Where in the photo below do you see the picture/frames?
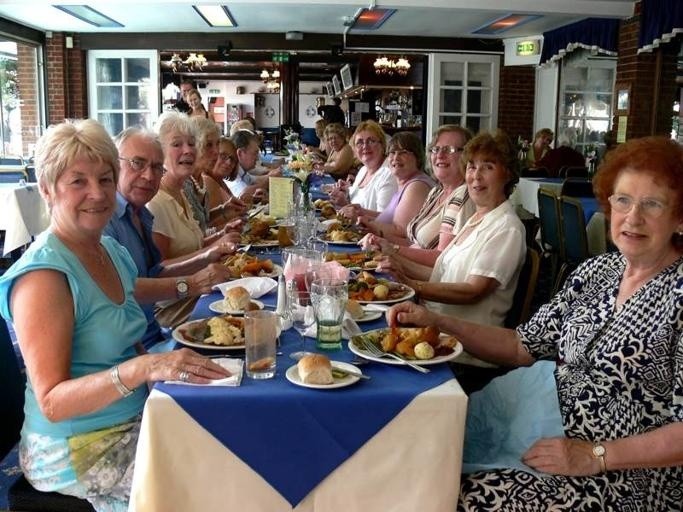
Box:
[613,82,632,116]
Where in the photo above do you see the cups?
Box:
[374,90,422,130]
[311,277,348,348]
[243,311,278,380]
[285,200,328,305]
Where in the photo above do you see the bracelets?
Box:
[109,363,137,399]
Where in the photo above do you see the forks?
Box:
[361,338,430,375]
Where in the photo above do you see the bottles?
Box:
[274,275,301,331]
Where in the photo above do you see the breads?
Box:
[298,354,335,386]
[223,286,251,311]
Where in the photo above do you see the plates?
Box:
[320,218,415,322]
[349,328,464,364]
[286,358,360,390]
[208,299,264,315]
[210,202,283,313]
[171,317,282,351]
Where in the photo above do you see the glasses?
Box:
[608,194,664,218]
[119,158,167,176]
[429,145,464,153]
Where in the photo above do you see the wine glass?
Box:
[288,292,318,351]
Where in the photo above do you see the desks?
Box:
[1,183,51,263]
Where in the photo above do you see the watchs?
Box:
[593,436,608,481]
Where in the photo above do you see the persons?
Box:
[148,76,284,329]
[382,136,683,511]
[517,127,555,170]
[357,126,530,394]
[294,97,527,328]
[102,122,232,355]
[1,116,234,512]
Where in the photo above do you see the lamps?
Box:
[373,54,412,77]
[260,64,280,93]
[169,53,207,73]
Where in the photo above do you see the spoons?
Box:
[289,350,371,380]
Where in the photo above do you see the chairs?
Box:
[1,317,98,512]
[1,153,38,183]
[505,164,614,331]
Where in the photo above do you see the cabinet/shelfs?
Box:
[329,83,423,139]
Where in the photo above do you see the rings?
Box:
[179,370,192,382]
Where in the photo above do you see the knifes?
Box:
[206,354,244,359]
